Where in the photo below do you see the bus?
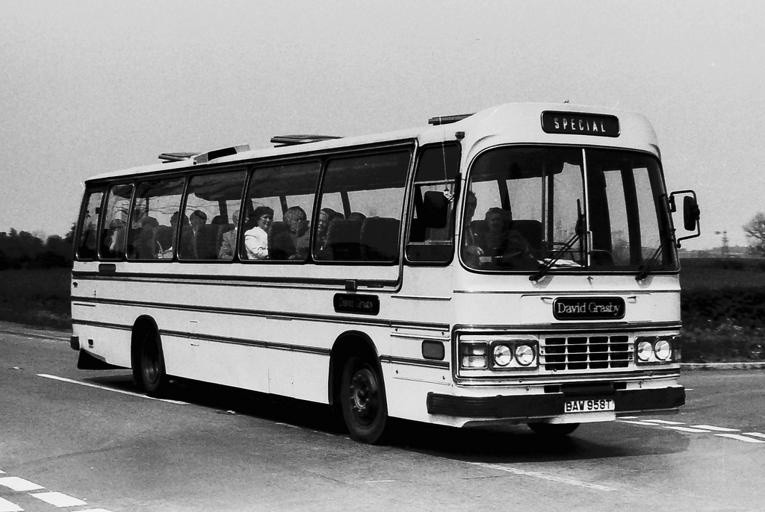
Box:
[67,99,702,448]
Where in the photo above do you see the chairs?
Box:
[102,211,544,262]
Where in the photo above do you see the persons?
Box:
[446,190,486,269]
[85,204,367,260]
[477,205,539,268]
